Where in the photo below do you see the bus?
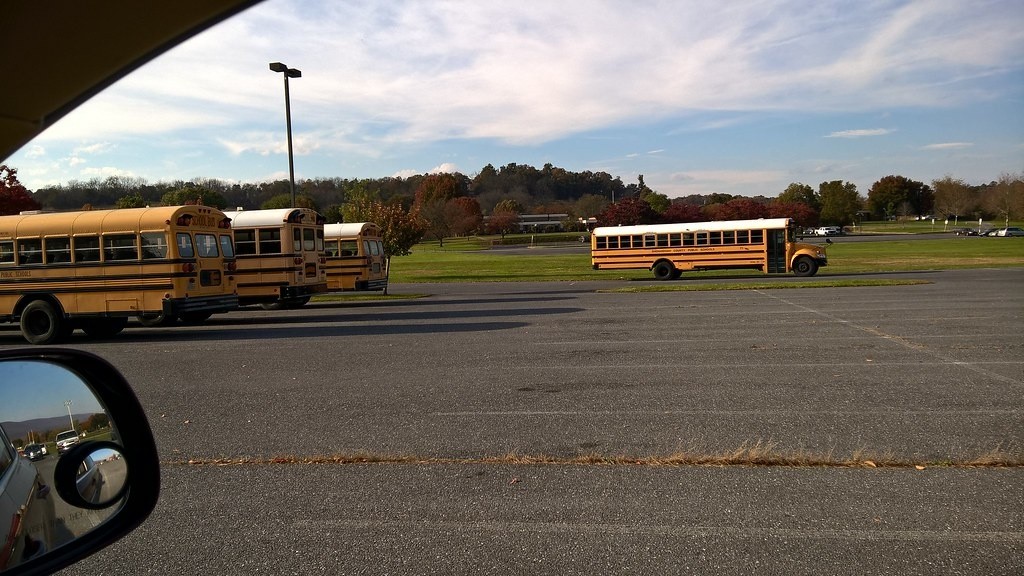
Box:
[590,217,833,280]
[217,207,329,308]
[1,204,238,347]
[322,221,388,293]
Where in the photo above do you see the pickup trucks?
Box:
[815,226,836,235]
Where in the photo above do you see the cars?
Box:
[802,226,816,235]
[578,236,590,243]
[914,214,931,221]
[52,429,81,455]
[830,226,847,236]
[23,444,43,462]
[39,444,50,455]
[998,227,1024,237]
[954,227,1000,237]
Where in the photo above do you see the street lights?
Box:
[269,61,301,208]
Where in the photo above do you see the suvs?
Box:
[944,213,962,221]
